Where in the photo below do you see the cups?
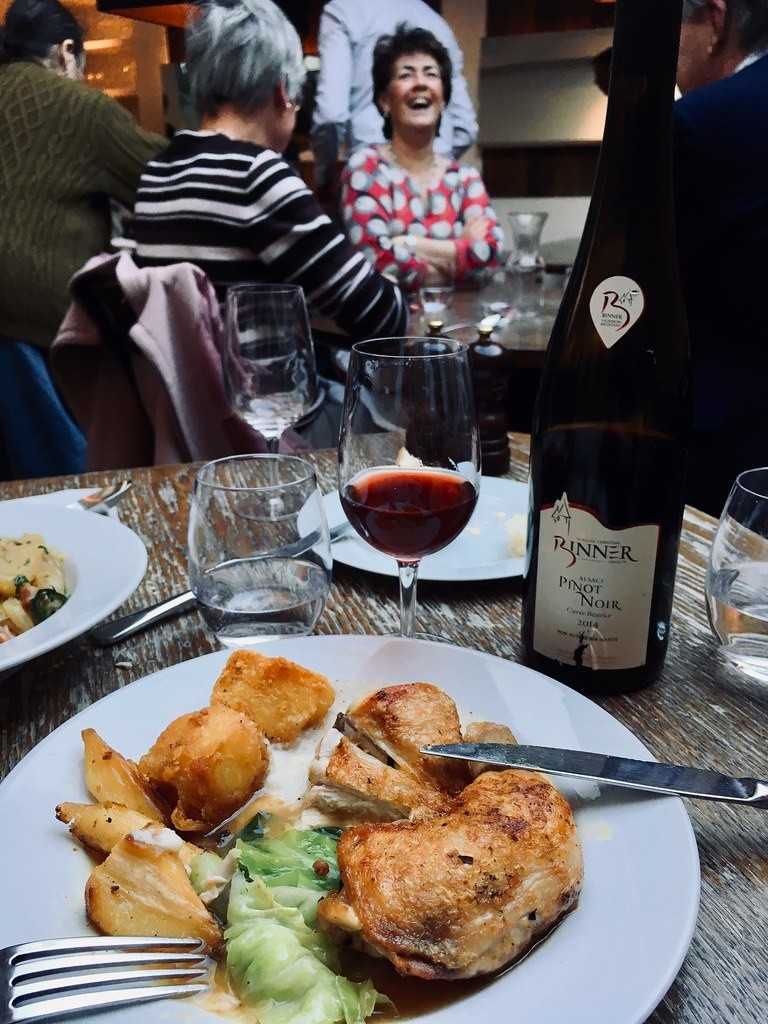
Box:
[187,453,331,643]
[419,286,448,324]
[705,465,768,690]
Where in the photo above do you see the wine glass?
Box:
[335,335,483,645]
[221,285,316,520]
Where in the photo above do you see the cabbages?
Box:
[187,828,403,1024]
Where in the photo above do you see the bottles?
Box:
[506,210,548,276]
[515,0,693,695]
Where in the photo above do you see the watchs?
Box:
[405,234,418,257]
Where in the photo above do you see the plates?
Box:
[296,469,542,583]
[1,498,148,677]
[0,640,699,1024]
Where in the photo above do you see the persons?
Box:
[0,0,172,481]
[613,0,768,537]
[132,0,410,450]
[340,19,505,294]
[309,0,481,237]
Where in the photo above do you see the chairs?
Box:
[95,268,227,463]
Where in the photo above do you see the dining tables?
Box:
[1,430,768,1024]
[305,269,575,421]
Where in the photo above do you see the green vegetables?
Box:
[12,541,72,624]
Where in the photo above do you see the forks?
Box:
[0,938,209,1024]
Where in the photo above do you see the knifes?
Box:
[416,742,768,823]
[65,479,133,511]
[87,522,352,647]
[204,526,322,574]
